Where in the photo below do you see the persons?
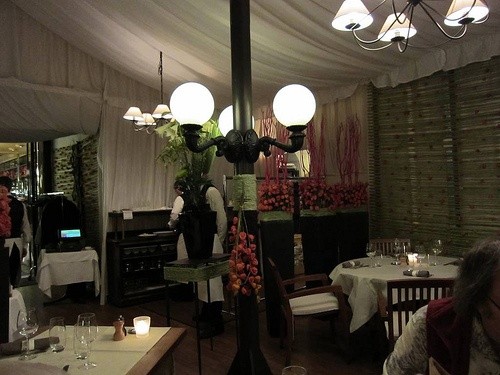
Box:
[0,174,31,281]
[383,239,500,375]
[168,177,229,336]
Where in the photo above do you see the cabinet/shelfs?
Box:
[105,208,181,305]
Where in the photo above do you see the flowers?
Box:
[256,104,368,214]
[227,216,264,302]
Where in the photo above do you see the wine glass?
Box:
[76,313,98,370]
[365,238,444,268]
[16,307,39,361]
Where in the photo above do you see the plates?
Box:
[138,231,175,237]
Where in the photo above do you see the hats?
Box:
[0,176,12,193]
[173,176,188,189]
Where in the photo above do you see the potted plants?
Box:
[154,119,221,259]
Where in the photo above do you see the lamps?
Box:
[169,81,317,164]
[122,50,172,134]
[331,0,490,53]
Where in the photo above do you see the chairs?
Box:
[384,277,462,353]
[369,238,410,255]
[266,256,352,370]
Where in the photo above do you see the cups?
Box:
[282,367,307,375]
[73,322,91,360]
[133,316,151,337]
[48,317,66,353]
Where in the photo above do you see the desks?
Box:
[162,252,233,375]
[37,247,100,304]
[0,321,189,375]
[328,256,465,334]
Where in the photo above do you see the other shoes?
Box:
[191,312,224,322]
[198,325,225,339]
